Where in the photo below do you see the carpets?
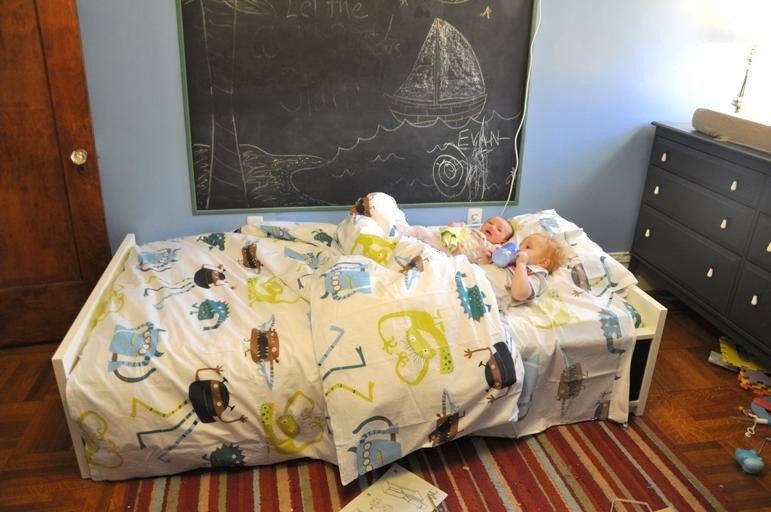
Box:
[92,412,736,512]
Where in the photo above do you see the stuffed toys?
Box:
[734,446,765,474]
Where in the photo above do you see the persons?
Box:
[409,215,514,265]
[478,230,567,311]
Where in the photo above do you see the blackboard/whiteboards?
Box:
[175,0,537,217]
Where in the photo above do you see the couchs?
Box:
[51,210,668,482]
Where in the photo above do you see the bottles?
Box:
[491,242,521,267]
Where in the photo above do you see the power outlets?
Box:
[467,207,482,224]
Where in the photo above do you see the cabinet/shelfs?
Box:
[629,121,771,369]
